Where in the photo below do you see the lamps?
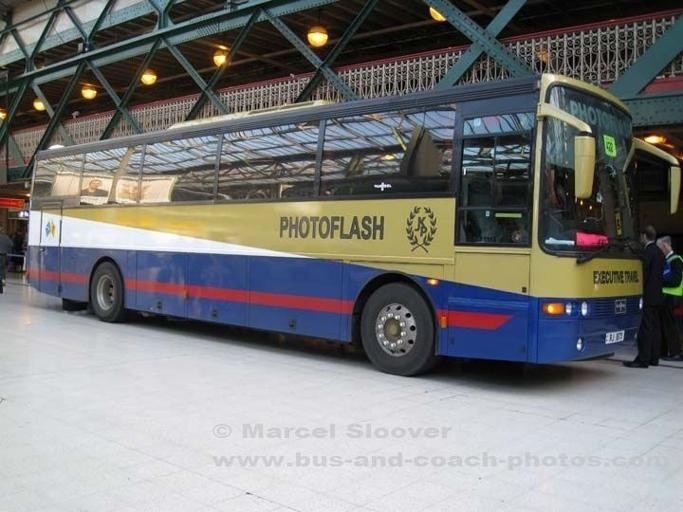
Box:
[213,37,230,67]
[308,8,329,47]
[140,68,157,86]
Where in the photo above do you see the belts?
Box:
[0,253,7,256]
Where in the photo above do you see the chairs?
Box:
[468,176,502,244]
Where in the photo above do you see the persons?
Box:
[623,225,664,368]
[656,236,683,361]
[0,223,14,294]
[80,179,108,197]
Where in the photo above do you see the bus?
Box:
[26,73,681,377]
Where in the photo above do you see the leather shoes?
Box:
[623,355,659,368]
[663,353,683,361]
[0,287,3,294]
